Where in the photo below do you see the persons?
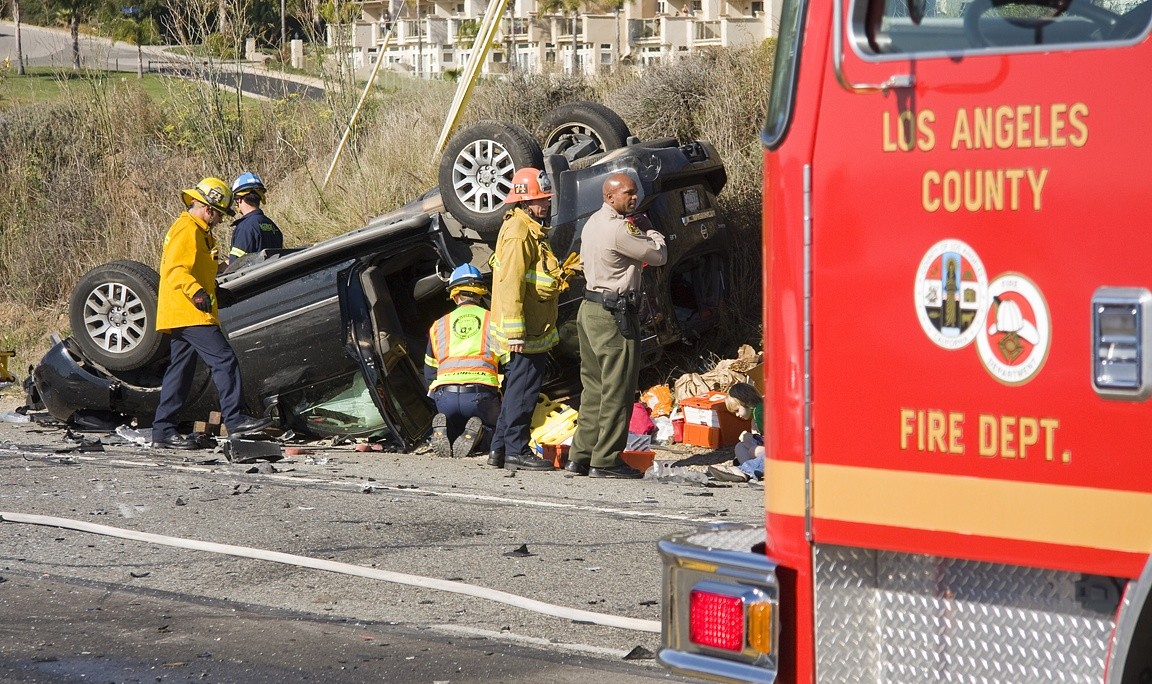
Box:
[488,166,567,470]
[153,175,275,450]
[566,171,668,480]
[421,259,502,461]
[706,382,764,481]
[231,172,288,272]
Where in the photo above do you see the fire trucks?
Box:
[665,2,1152,682]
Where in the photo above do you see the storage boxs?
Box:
[679,391,752,450]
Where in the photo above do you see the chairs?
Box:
[410,268,454,315]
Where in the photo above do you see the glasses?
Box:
[215,209,225,217]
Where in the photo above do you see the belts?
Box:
[584,290,603,304]
[442,385,490,393]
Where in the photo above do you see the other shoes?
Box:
[451,416,485,459]
[432,413,454,458]
[708,464,750,482]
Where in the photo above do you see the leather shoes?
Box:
[565,460,589,474]
[487,450,505,466]
[228,415,274,440]
[503,451,555,471]
[152,433,199,450]
[588,462,644,479]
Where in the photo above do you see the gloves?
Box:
[215,287,235,309]
[193,288,212,313]
[557,252,583,293]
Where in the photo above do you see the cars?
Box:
[33,104,733,449]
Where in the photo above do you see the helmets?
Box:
[229,171,267,200]
[505,168,556,203]
[178,177,236,224]
[446,263,486,293]
